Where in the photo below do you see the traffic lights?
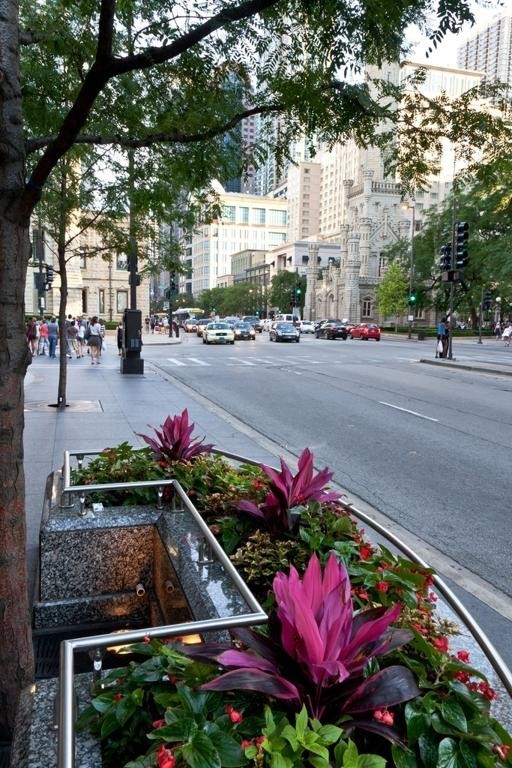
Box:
[439,246,451,268]
[408,290,416,307]
[169,271,176,286]
[43,265,54,292]
[483,289,491,312]
[290,283,301,306]
[455,222,469,268]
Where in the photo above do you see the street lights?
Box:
[402,203,415,338]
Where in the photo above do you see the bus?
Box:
[151,307,205,326]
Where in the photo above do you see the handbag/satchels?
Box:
[84,328,90,339]
[438,340,443,353]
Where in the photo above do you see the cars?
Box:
[265,313,380,342]
[184,316,264,344]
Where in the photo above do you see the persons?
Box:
[494,320,512,347]
[438,318,448,358]
[144,313,170,334]
[444,321,449,357]
[116,321,123,357]
[25,314,105,365]
